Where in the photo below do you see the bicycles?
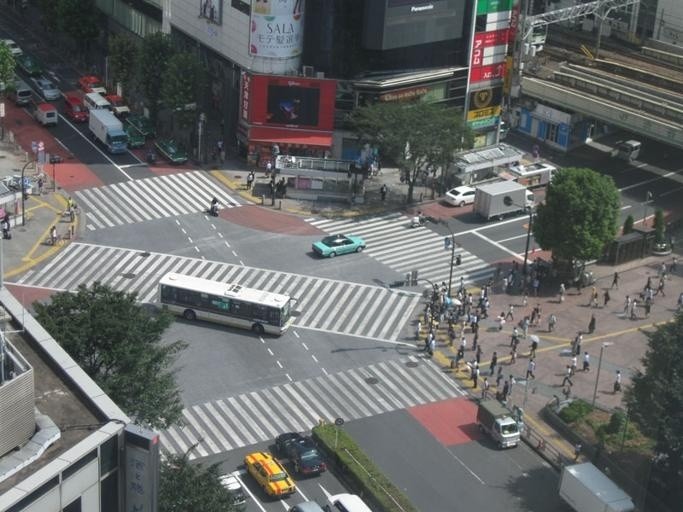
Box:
[46,234,65,246]
[61,207,81,217]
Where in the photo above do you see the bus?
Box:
[156,271,299,336]
[618,140,645,162]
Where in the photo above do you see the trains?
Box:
[517,14,549,53]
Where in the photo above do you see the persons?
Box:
[515,406,524,420]
[204,141,286,210]
[0,178,75,247]
[416,235,683,405]
[200,1,217,22]
[573,439,582,460]
[380,183,387,200]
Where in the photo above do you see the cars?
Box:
[444,185,477,207]
[65,75,131,121]
[2,38,61,105]
[312,233,367,258]
[243,451,297,499]
[123,114,188,163]
[276,432,328,478]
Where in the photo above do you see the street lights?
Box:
[425,215,455,298]
[505,200,532,275]
[22,153,57,226]
[390,278,436,338]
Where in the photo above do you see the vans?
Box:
[291,492,373,512]
[34,103,57,125]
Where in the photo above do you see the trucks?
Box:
[499,162,559,189]
[474,180,535,220]
[558,461,640,512]
[477,399,521,448]
[88,109,128,155]
